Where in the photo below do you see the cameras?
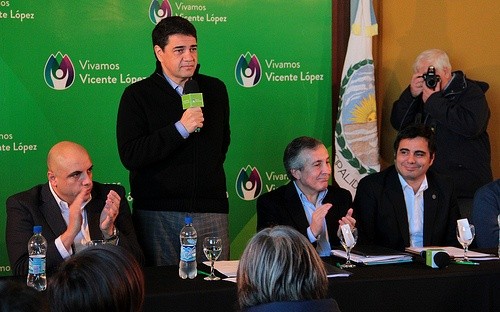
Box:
[418,65,440,91]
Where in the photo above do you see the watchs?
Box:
[100,226,119,241]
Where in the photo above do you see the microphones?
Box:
[182,78,204,133]
[419,249,451,268]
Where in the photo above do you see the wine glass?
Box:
[456,224,476,264]
[339,227,358,267]
[203,237,222,280]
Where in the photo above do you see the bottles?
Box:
[178,216,197,279]
[26,226,47,292]
[497,214,500,258]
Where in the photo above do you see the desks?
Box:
[0,248,500,312]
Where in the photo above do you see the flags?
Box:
[335,0,383,200]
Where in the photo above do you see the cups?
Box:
[88,240,104,248]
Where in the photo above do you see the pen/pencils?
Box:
[336,262,343,269]
[452,261,481,265]
[197,268,211,276]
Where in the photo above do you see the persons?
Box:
[255,137,361,257]
[46,245,145,312]
[235,225,340,312]
[117,16,232,267]
[473,180,499,253]
[350,128,470,253]
[389,49,494,244]
[4,140,147,280]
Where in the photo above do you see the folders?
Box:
[330,244,417,266]
